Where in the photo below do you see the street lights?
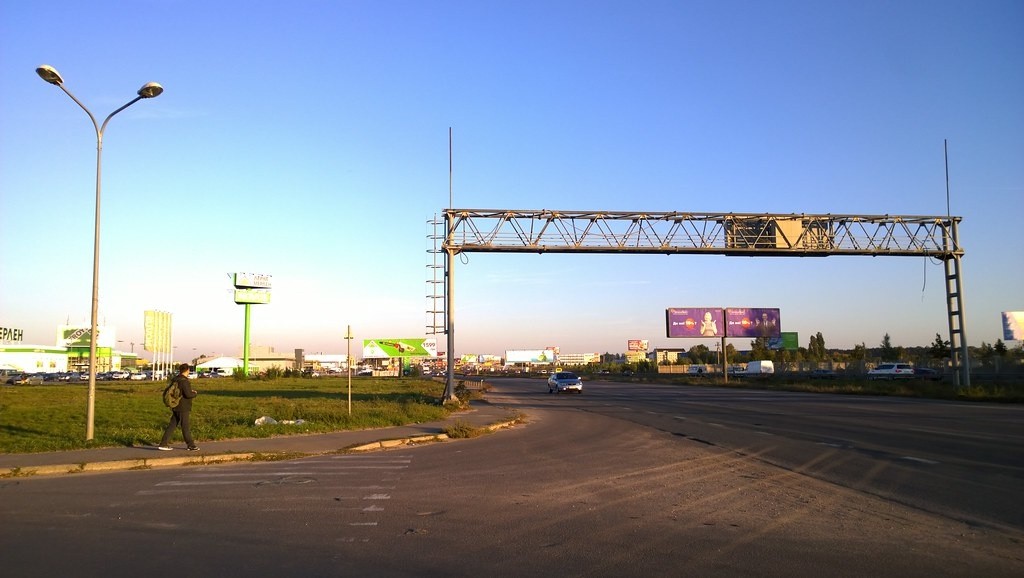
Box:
[36,65,164,440]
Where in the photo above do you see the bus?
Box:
[551,367,562,373]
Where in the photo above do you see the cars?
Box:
[546,371,583,394]
[514,370,520,374]
[210,373,219,378]
[15,372,43,386]
[39,370,179,382]
[424,370,432,375]
[726,366,744,374]
[867,364,913,381]
[203,371,212,377]
[809,369,835,380]
[305,371,320,377]
[187,372,197,379]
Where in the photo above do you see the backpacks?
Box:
[163,378,188,408]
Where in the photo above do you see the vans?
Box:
[686,366,706,373]
[537,369,546,374]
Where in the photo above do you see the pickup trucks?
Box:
[217,369,225,377]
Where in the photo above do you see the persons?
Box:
[700,312,717,336]
[755,313,776,337]
[158,363,201,451]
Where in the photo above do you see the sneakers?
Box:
[187,445,200,451]
[157,442,174,450]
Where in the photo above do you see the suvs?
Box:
[463,371,471,377]
[0,369,17,385]
[598,370,609,376]
[622,369,634,376]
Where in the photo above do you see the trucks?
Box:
[745,360,774,375]
